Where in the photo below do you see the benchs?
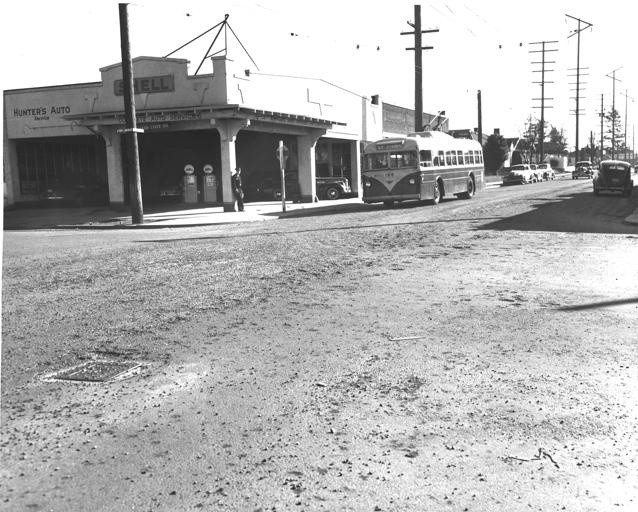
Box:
[160,188,183,200]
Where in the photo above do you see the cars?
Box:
[502,162,558,185]
[571,160,595,180]
[256,170,351,201]
[592,159,635,197]
[634,160,638,174]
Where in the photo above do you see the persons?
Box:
[232,167,245,211]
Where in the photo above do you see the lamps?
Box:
[209,108,216,126]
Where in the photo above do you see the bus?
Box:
[360,131,486,206]
